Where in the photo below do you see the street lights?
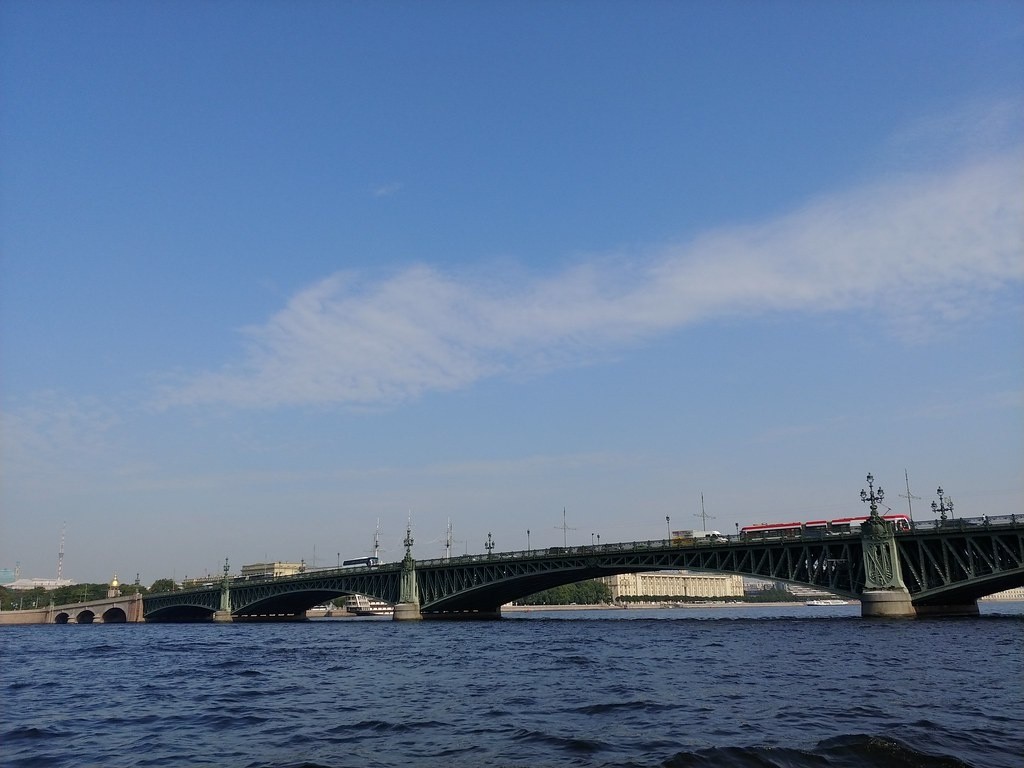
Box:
[736,523,738,535]
[666,514,672,550]
[527,528,530,558]
[592,532,600,546]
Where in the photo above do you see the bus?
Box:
[740,514,913,541]
[343,556,379,567]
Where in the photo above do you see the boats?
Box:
[806,594,849,606]
